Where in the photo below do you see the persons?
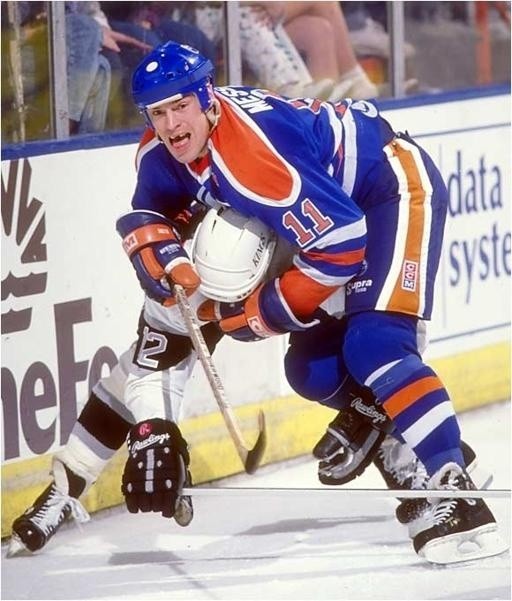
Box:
[116,37,500,531]
[8,206,477,554]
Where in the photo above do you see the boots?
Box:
[12,461,85,553]
[373,431,497,554]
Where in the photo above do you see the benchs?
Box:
[1,22,390,144]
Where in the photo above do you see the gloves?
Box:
[116,209,202,307]
[197,272,322,342]
[121,418,193,519]
[313,387,397,485]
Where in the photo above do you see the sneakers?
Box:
[277,78,356,103]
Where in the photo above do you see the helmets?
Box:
[132,40,217,129]
[190,206,278,303]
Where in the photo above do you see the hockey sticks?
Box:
[173,284,267,476]
